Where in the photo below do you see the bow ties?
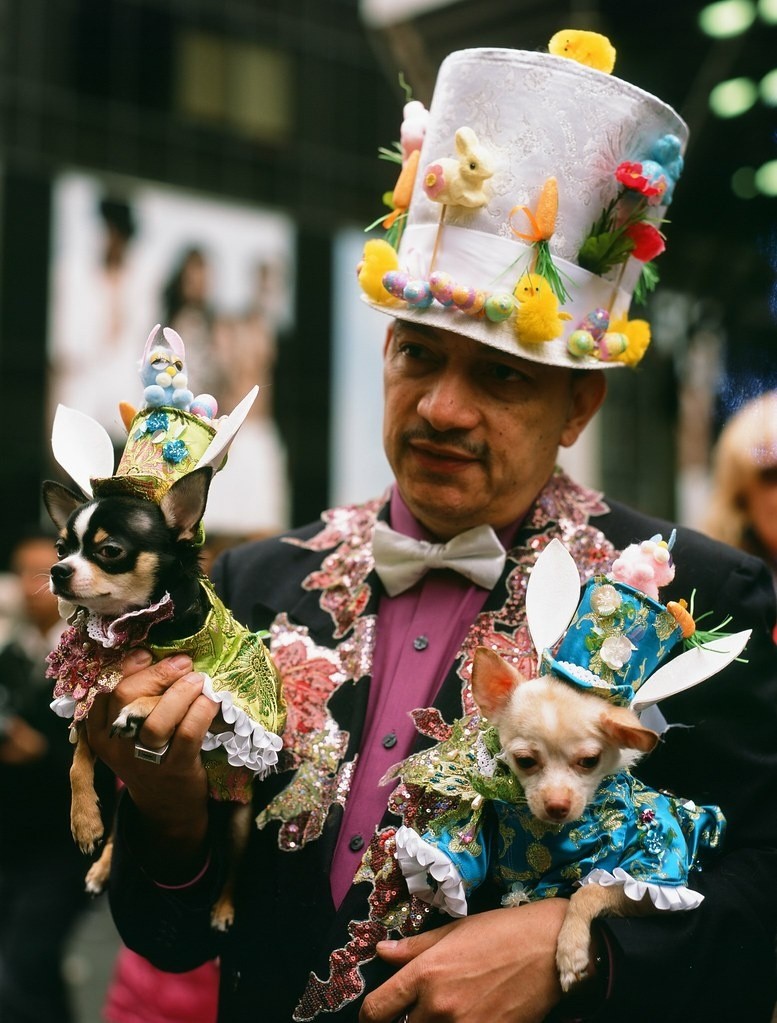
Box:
[370,522,507,598]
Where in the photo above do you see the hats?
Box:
[357,29,690,369]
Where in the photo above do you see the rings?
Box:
[133,741,170,765]
[403,1012,409,1023]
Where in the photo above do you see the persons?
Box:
[52,199,279,420]
[0,538,119,1023]
[85,47,777,1023]
[709,388,777,570]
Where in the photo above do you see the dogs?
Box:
[43,463,268,936]
[427,644,689,992]
